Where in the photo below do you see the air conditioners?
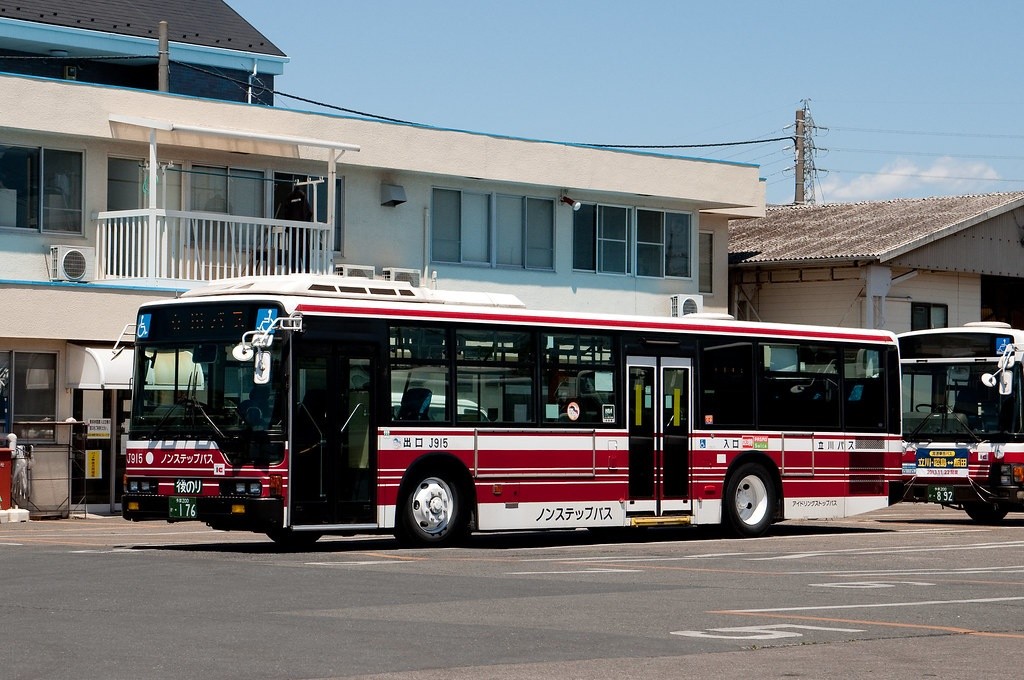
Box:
[670,294,703,317]
[383,267,420,288]
[49,244,96,283]
[335,264,375,280]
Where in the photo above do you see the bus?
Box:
[861,319,1024,523]
[109,271,903,549]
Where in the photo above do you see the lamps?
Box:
[561,196,581,210]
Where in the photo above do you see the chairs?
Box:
[398,388,432,419]
[952,387,986,415]
[238,385,271,418]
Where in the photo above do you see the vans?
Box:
[390,391,492,422]
